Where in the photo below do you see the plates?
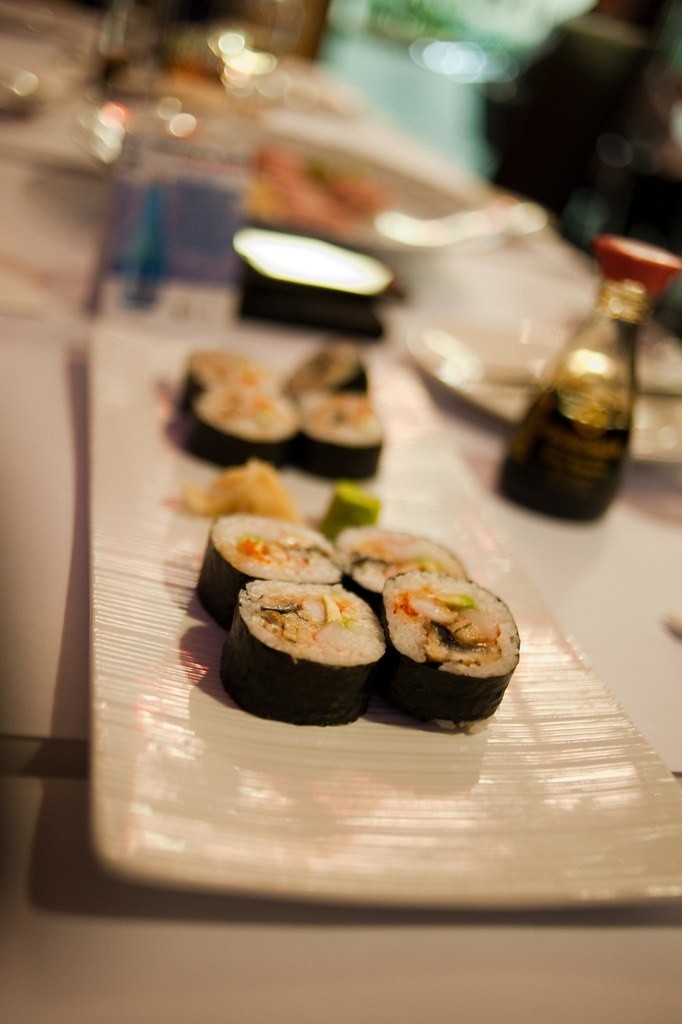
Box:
[375,304,682,467]
[78,325,682,916]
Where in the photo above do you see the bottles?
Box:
[492,235,682,521]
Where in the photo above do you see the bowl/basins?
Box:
[230,227,390,338]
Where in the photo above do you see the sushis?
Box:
[173,342,383,480]
[197,510,522,726]
